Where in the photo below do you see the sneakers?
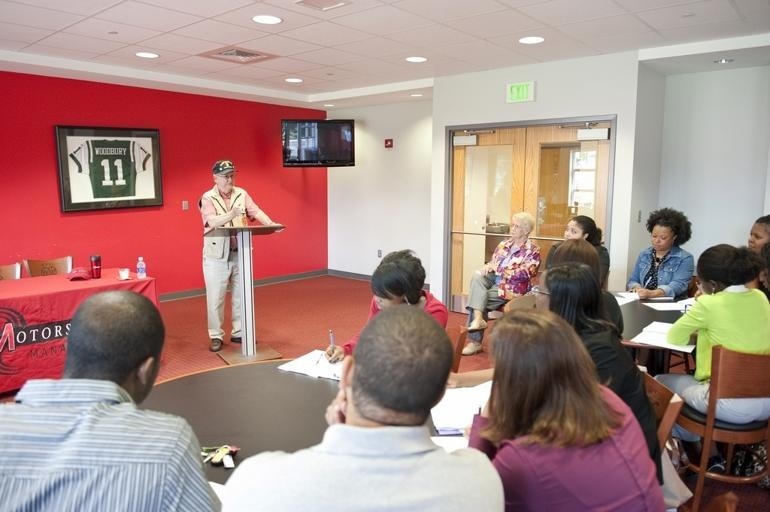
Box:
[688,455,727,486]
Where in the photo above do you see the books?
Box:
[273,346,350,383]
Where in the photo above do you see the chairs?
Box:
[675,344,770,511]
[0,256,72,281]
[643,372,685,470]
[444,323,468,376]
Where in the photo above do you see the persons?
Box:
[635,210,769,489]
[460,211,609,356]
[325,249,448,363]
[466,306,668,512]
[0,288,225,512]
[219,302,507,511]
[196,158,287,353]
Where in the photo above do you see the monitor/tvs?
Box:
[281,119,355,167]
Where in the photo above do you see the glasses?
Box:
[531,286,550,297]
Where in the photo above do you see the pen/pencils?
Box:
[329,330,334,357]
[643,276,652,288]
[684,305,687,313]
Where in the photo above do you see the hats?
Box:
[68,267,92,281]
[212,160,239,174]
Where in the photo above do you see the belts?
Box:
[230,246,238,251]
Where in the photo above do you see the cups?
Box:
[90,256,102,279]
[119,268,129,279]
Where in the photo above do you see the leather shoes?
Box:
[467,319,489,333]
[462,343,483,356]
[209,338,223,352]
[231,337,242,342]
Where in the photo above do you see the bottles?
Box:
[136,257,147,279]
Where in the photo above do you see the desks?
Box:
[0,267,164,396]
[135,357,341,507]
[608,290,696,375]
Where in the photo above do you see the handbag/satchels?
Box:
[731,442,770,490]
